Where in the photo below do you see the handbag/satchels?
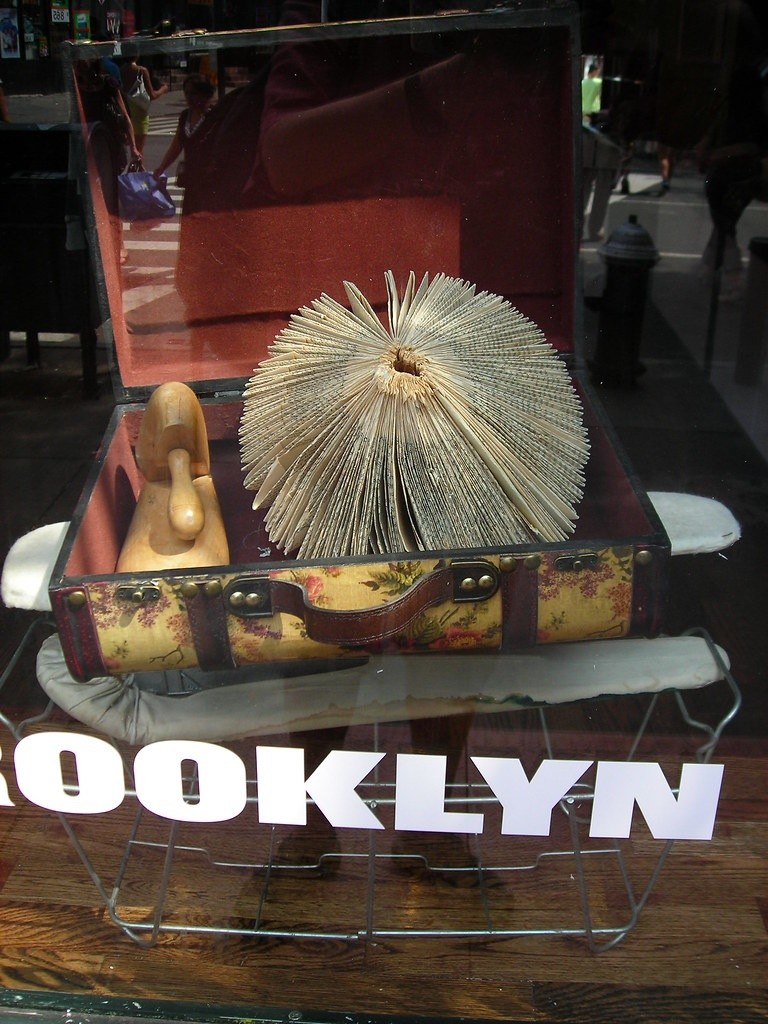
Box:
[118,159,175,222]
[127,66,150,118]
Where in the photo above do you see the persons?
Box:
[0,1,676,382]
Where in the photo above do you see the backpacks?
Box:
[78,73,123,141]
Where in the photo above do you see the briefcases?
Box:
[51,8,671,682]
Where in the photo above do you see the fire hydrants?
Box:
[580,213,664,390]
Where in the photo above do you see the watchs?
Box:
[403,72,449,141]
